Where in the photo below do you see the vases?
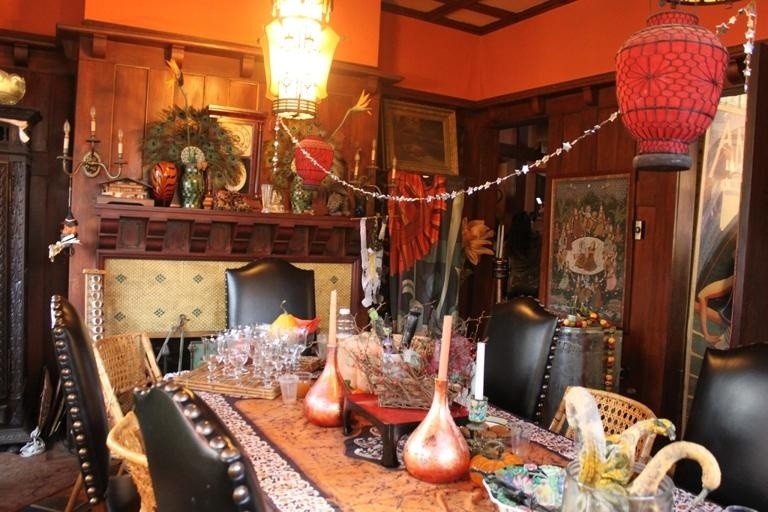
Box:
[400,377,471,486]
[147,158,176,209]
[289,174,311,217]
[176,163,209,212]
[301,346,352,428]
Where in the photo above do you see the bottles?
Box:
[335,308,357,345]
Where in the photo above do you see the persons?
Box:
[556,202,620,307]
[693,248,736,347]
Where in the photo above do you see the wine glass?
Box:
[200,323,309,390]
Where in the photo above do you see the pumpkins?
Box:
[468,447,524,487]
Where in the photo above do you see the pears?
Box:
[268,313,302,333]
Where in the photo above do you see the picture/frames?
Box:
[374,96,460,179]
[536,165,637,335]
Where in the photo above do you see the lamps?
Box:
[260,0,341,128]
[54,102,130,183]
[344,135,399,200]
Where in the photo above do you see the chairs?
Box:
[90,328,161,427]
[668,336,767,512]
[130,375,267,510]
[471,291,562,436]
[217,256,321,360]
[46,291,144,512]
[545,383,661,483]
[103,407,158,511]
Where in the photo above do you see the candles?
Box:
[327,287,343,349]
[435,311,456,381]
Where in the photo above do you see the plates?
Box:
[223,160,247,192]
[482,461,568,511]
[215,121,250,154]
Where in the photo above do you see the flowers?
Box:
[166,56,205,168]
[290,88,374,175]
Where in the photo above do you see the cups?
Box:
[562,460,678,511]
[277,373,299,403]
[262,184,275,213]
[294,370,312,396]
[508,422,532,456]
